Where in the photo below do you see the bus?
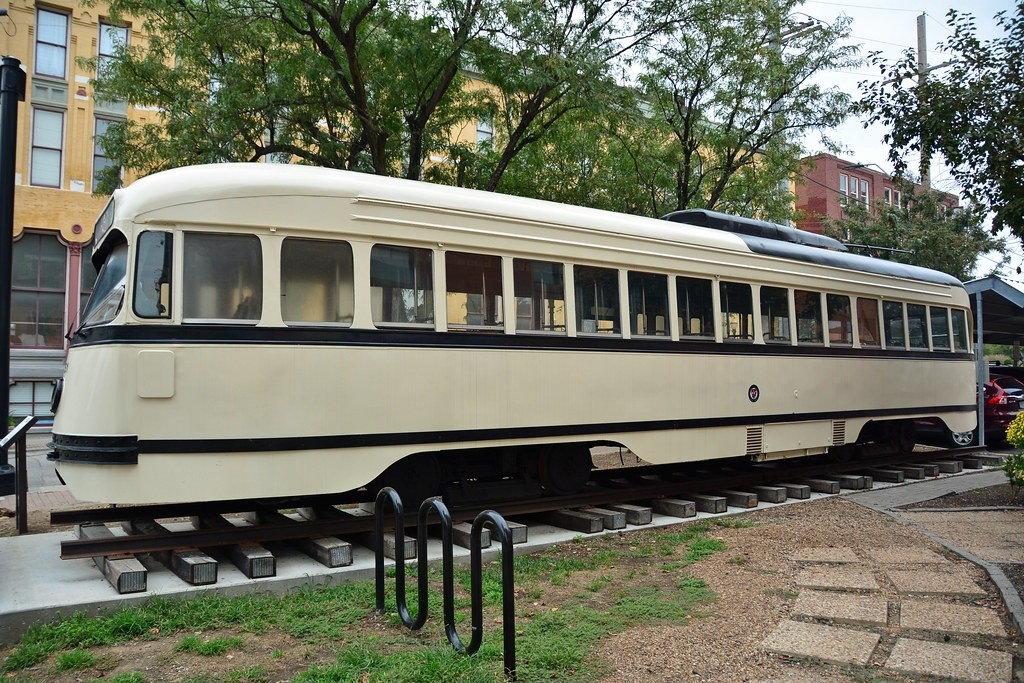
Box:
[44,160,978,560]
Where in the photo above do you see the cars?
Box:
[989,363,1024,385]
[913,373,1024,447]
